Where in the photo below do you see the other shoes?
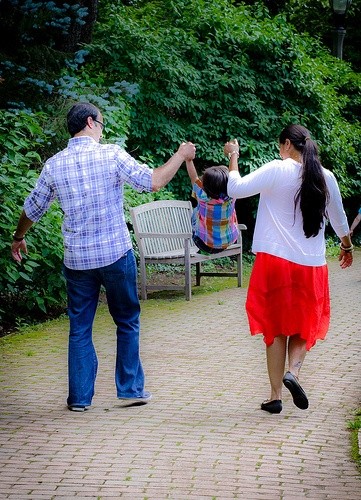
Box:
[68,404,85,411]
[262,400,283,414]
[120,392,152,401]
[283,372,309,410]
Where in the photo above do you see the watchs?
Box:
[228,151,239,160]
[13,231,25,241]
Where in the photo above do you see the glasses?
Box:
[92,118,106,131]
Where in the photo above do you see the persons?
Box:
[185,160,240,254]
[224,125,354,413]
[349,208,361,237]
[12,102,196,411]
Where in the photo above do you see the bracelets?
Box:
[340,242,353,253]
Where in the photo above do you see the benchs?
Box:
[130,200,247,301]
